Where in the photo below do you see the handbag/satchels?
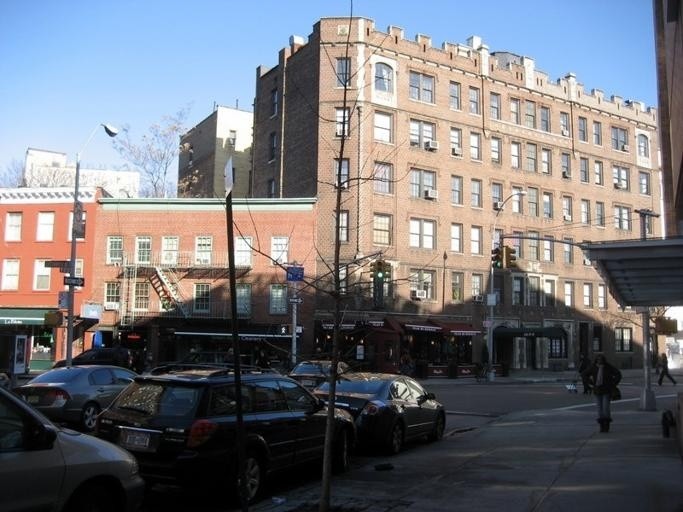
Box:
[610,386,621,400]
[592,384,610,394]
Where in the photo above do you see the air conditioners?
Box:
[472,294,483,301]
[492,201,506,209]
[425,140,439,150]
[410,288,425,299]
[623,144,630,150]
[424,189,438,198]
[562,129,570,136]
[562,171,572,178]
[453,147,462,155]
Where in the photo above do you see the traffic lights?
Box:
[368,262,375,279]
[376,261,382,279]
[490,248,501,268]
[509,249,516,268]
[384,262,392,279]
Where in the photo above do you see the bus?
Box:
[89,325,302,370]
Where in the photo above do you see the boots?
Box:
[596,417,614,432]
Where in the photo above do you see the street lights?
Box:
[64,122,119,368]
[486,190,527,383]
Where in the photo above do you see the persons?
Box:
[254,344,259,363]
[656,353,676,387]
[126,349,133,369]
[399,348,415,376]
[576,352,593,397]
[224,347,234,362]
[581,353,622,433]
[254,350,270,368]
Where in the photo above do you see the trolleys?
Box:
[565,369,579,394]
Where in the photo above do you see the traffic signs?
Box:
[288,298,304,304]
[44,259,71,268]
[64,276,83,288]
